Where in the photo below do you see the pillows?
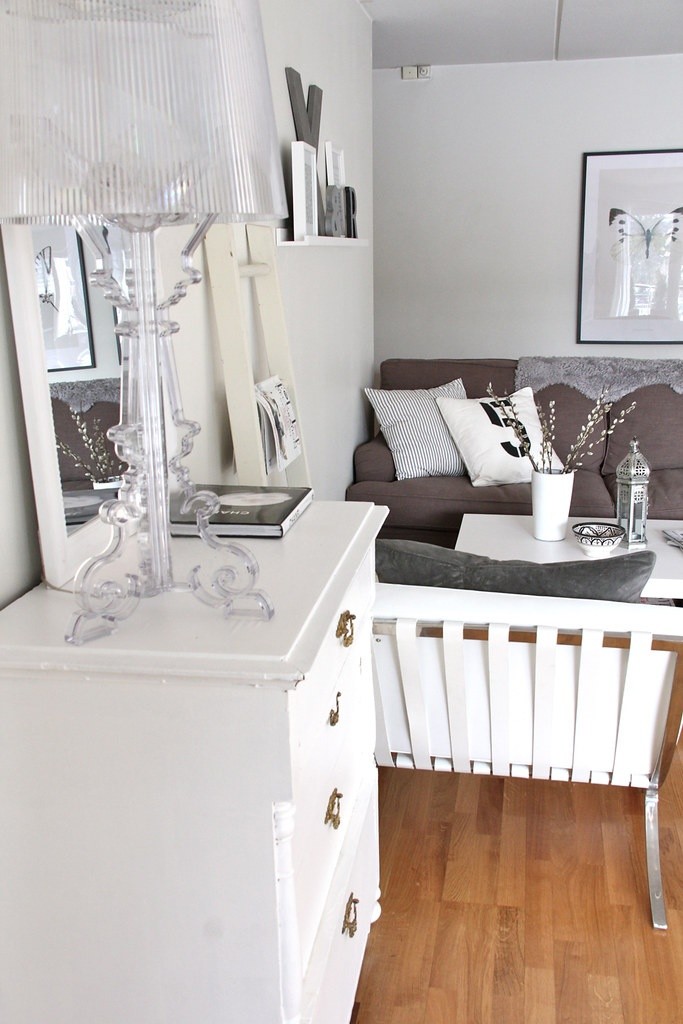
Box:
[435,387,565,487]
[373,539,656,604]
[364,377,469,482]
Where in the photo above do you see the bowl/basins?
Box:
[571,522,626,557]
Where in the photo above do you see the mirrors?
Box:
[0,221,124,587]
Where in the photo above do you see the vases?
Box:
[531,469,574,542]
[92,476,122,490]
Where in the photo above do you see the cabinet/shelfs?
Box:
[0,500,390,1024]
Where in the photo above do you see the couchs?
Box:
[345,356,683,549]
[48,378,120,490]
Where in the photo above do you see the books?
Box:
[169,484,313,540]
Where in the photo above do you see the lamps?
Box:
[0,0,289,646]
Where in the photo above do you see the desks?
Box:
[455,512,683,599]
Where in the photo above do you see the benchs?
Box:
[371,581,683,927]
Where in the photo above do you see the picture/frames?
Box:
[576,149,683,344]
[29,220,96,372]
[291,141,317,242]
[326,142,345,188]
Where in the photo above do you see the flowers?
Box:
[486,382,637,474]
[56,395,123,482]
